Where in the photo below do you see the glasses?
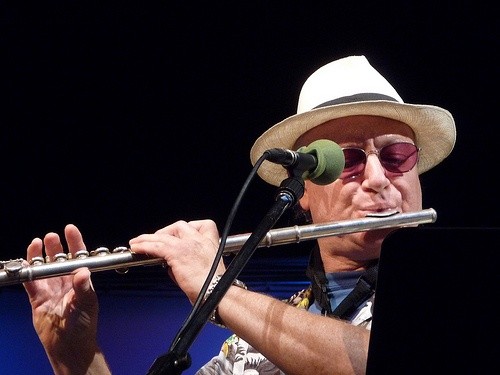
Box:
[342,143,421,180]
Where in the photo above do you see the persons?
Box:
[23,54,422,375]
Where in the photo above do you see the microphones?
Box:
[263,139,345,185]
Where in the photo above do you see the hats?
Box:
[250,56,456,188]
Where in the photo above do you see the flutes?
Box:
[0,207,436,287]
